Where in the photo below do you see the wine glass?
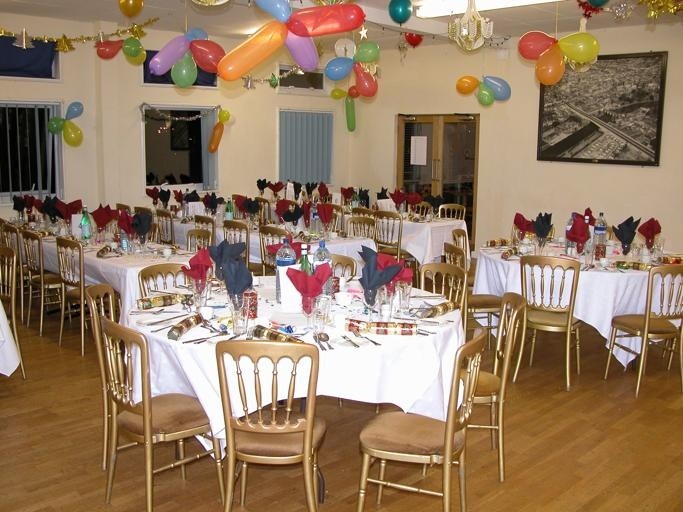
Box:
[432,206,439,221]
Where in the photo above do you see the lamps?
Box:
[414,0,553,19]
[447,0,493,51]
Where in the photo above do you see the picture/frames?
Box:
[536,49,667,167]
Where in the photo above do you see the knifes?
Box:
[312,333,326,350]
[341,334,359,348]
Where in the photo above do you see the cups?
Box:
[415,214,420,220]
[511,224,665,266]
[192,279,412,334]
[331,232,337,240]
[426,214,431,222]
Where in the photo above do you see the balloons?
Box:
[119,0,144,17]
[389,1,413,25]
[48,102,83,146]
[404,32,424,48]
[209,111,230,151]
[217,0,380,131]
[455,76,511,105]
[518,31,600,86]
[149,28,225,88]
[98,36,147,67]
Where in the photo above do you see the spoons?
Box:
[319,333,334,350]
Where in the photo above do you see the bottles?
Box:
[352,187,358,207]
[81,205,91,239]
[310,205,318,234]
[565,212,607,260]
[225,197,233,220]
[275,238,332,304]
[120,231,127,251]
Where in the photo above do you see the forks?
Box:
[352,330,381,345]
